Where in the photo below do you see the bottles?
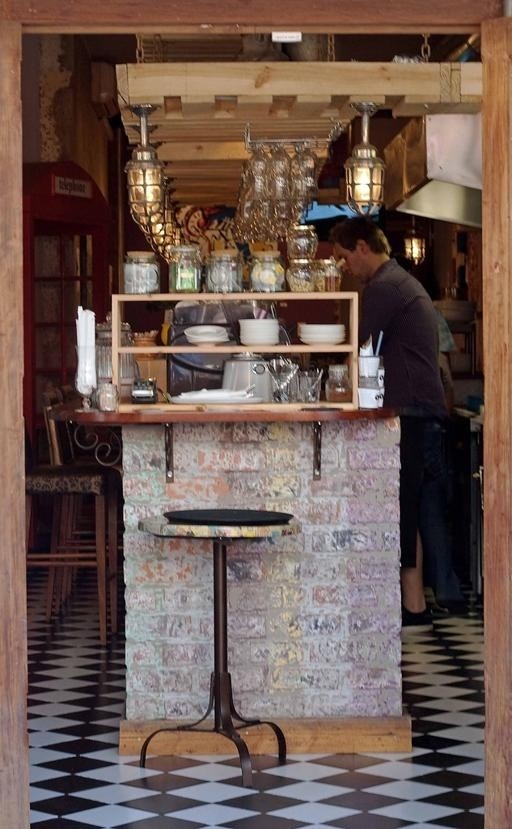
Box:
[207,250,245,294]
[325,364,353,403]
[122,251,162,294]
[167,247,202,294]
[317,259,342,292]
[286,225,319,260]
[286,258,317,292]
[249,250,286,293]
[94,310,136,405]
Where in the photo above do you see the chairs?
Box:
[40,384,124,625]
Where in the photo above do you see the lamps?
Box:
[344,103,386,216]
[122,102,183,263]
[404,213,428,267]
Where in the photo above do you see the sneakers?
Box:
[402,596,448,627]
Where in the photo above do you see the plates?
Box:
[170,389,264,406]
[238,318,281,347]
[183,324,231,346]
[299,323,347,346]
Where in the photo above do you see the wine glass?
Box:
[232,136,320,245]
[72,369,100,414]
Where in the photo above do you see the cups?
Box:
[357,356,386,412]
[97,383,119,415]
[269,372,321,405]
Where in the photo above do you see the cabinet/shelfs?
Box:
[439,323,475,381]
[111,291,360,410]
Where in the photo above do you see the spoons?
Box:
[264,359,323,402]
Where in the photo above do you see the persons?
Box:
[326,217,470,630]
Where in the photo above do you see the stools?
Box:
[137,511,295,790]
[25,463,118,644]
